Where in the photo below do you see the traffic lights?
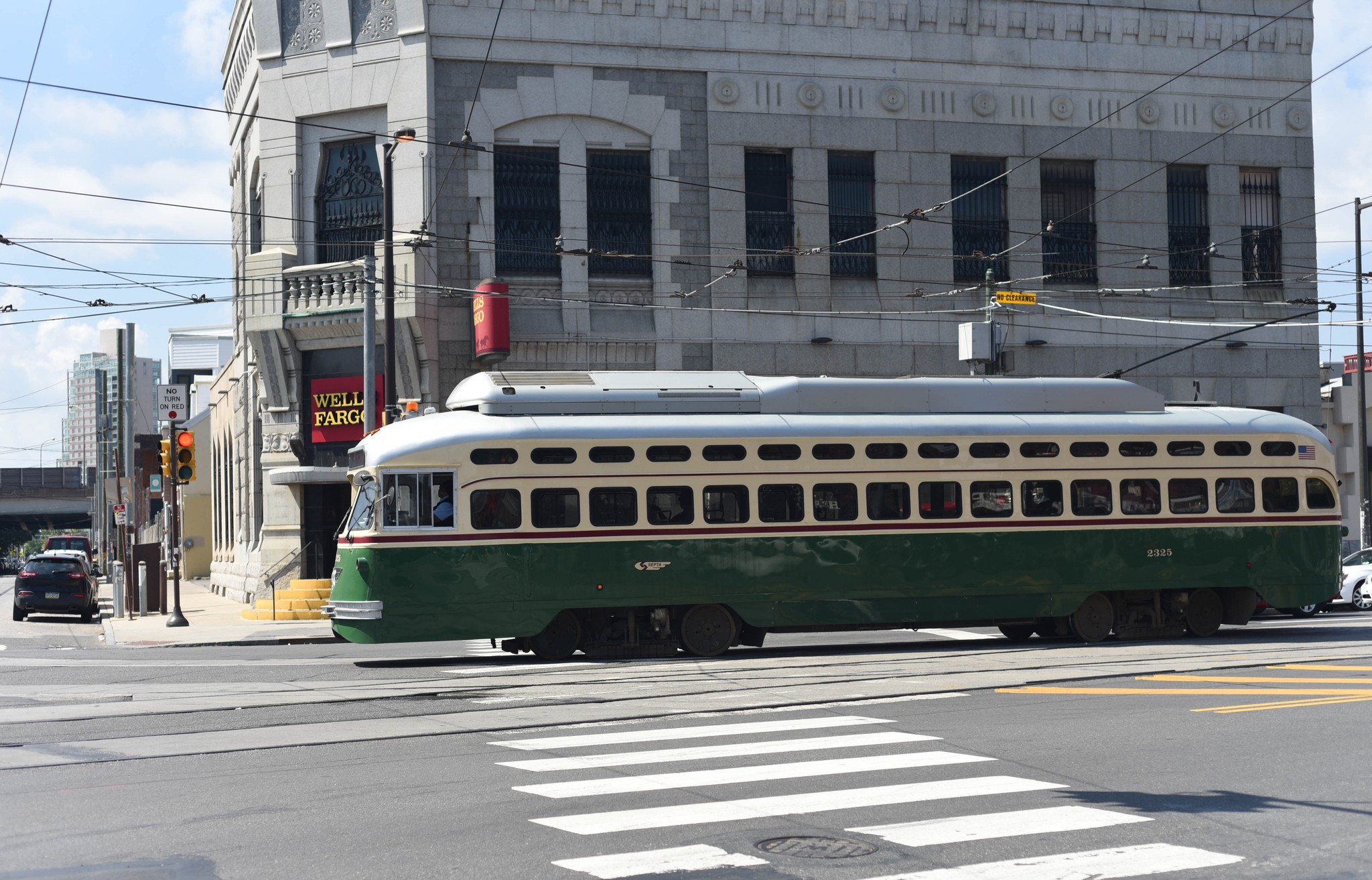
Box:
[157,439,172,478]
[177,431,197,481]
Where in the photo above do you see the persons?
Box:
[399,479,454,527]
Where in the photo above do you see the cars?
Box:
[0,558,26,573]
[1276,547,1372,618]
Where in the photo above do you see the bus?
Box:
[322,268,1350,660]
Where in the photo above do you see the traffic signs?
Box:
[114,505,127,525]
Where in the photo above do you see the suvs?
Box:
[44,535,98,567]
[13,552,100,623]
[43,549,99,577]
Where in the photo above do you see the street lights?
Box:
[382,124,415,418]
